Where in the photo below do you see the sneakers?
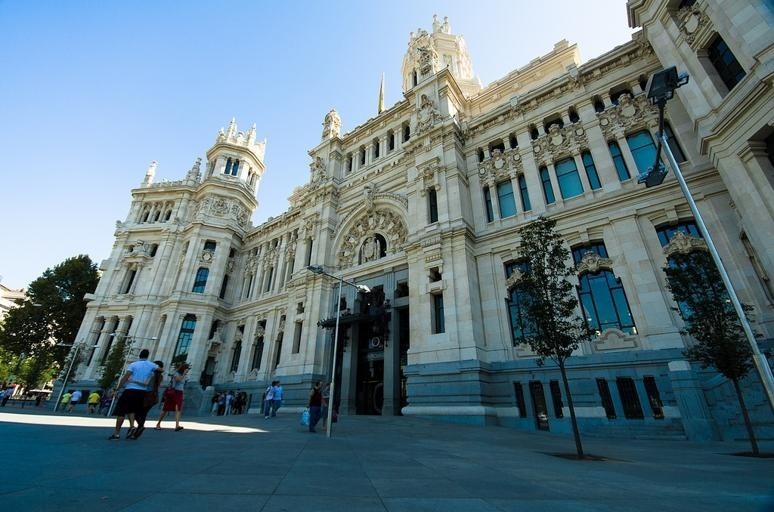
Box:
[110,426,145,439]
[175,426,183,431]
[156,424,161,429]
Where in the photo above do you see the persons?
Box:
[320,383,337,430]
[271,380,282,416]
[155,363,189,431]
[262,380,277,419]
[308,380,321,432]
[127,360,163,439]
[107,348,164,441]
[86,389,115,416]
[65,388,81,414]
[58,389,71,412]
[207,391,246,416]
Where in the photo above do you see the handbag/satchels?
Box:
[300,411,310,426]
[154,393,160,406]
[333,416,337,422]
[162,389,176,402]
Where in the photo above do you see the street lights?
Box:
[306,266,373,438]
[12,352,25,375]
[105,332,155,419]
[636,65,774,411]
[52,341,97,414]
